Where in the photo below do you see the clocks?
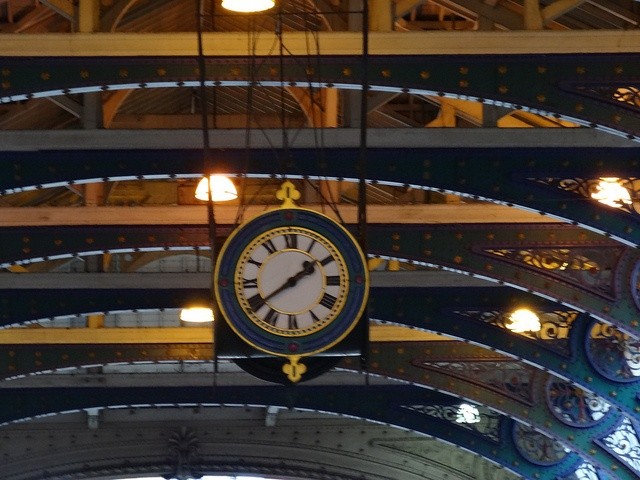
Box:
[210,182,369,381]
